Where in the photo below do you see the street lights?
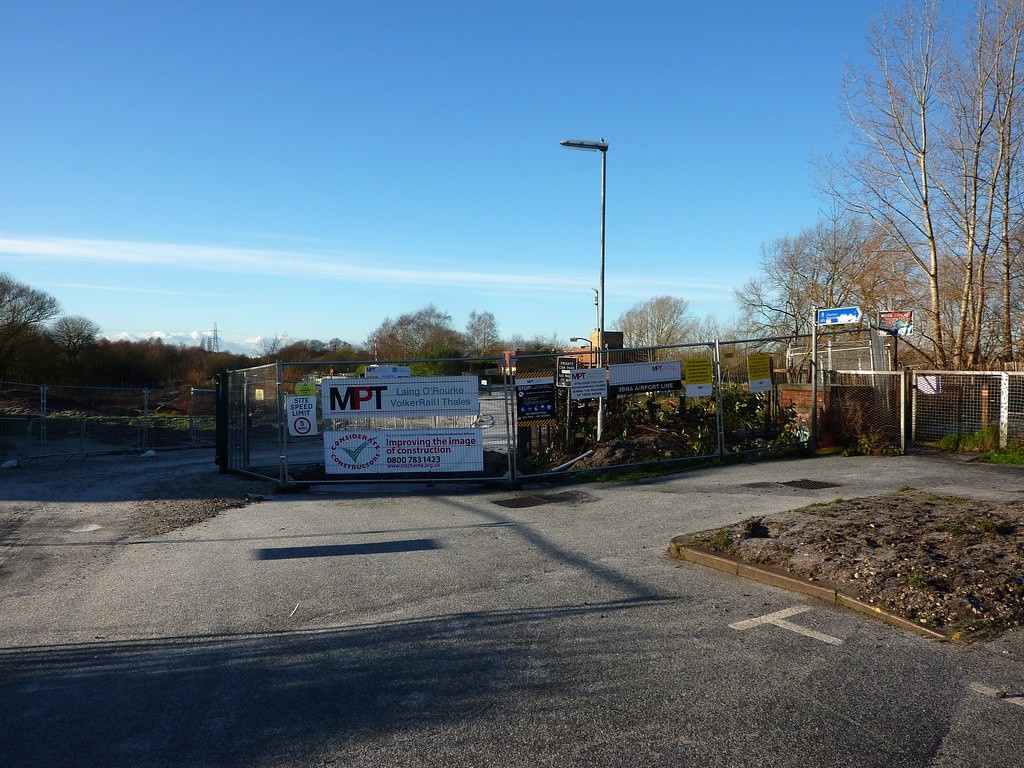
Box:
[374,338,377,361]
[570,337,592,368]
[591,288,599,368]
[559,138,611,368]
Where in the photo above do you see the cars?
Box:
[343,372,355,376]
[718,429,769,442]
[656,386,672,397]
[314,372,322,378]
[272,413,287,429]
[155,403,188,415]
[112,404,142,415]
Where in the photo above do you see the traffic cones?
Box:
[816,407,845,454]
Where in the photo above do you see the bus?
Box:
[7,390,32,401]
